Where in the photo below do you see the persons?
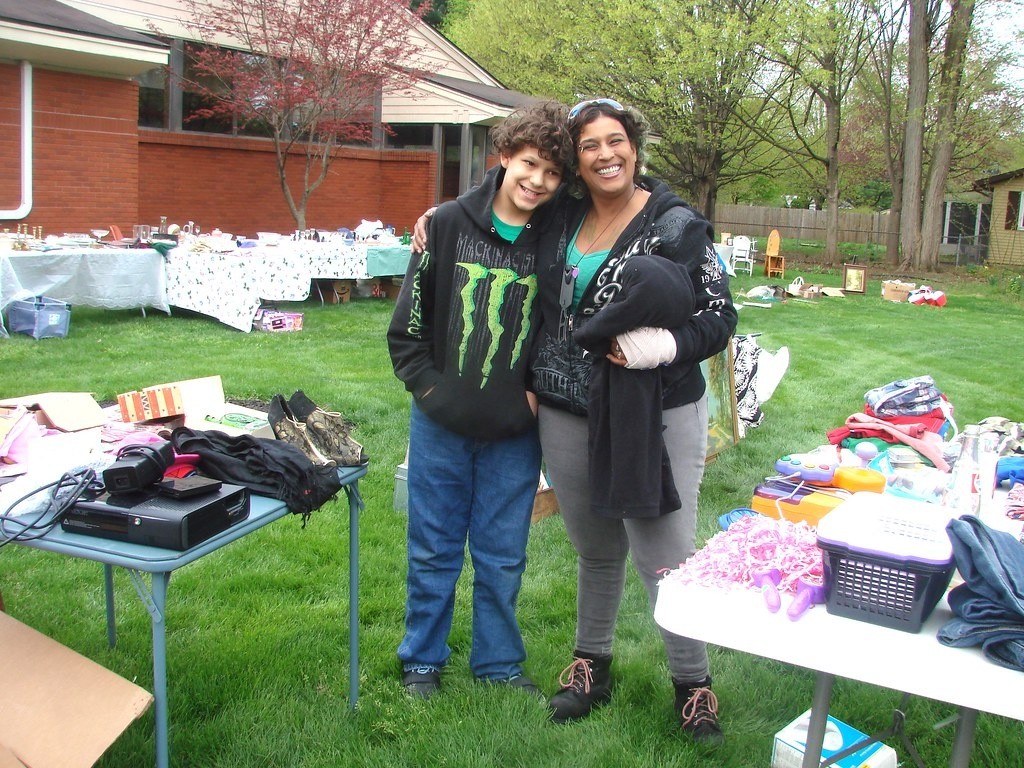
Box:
[387,106,579,699]
[410,103,738,745]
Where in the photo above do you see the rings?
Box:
[616,345,620,352]
[617,352,622,359]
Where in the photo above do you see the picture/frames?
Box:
[704,335,739,469]
[842,264,867,295]
[880,282,915,302]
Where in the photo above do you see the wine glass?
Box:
[290,228,346,243]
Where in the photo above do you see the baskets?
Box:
[815,490,955,634]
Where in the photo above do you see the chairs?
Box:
[730,235,753,278]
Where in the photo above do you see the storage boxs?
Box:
[7,295,72,341]
[384,281,401,299]
[1,610,155,768]
[770,707,897,768]
[311,279,353,304]
[116,374,272,437]
[252,309,304,331]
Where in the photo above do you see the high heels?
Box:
[266,393,337,476]
[287,389,371,467]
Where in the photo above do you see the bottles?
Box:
[981,430,997,518]
[159,217,168,234]
[188,221,194,235]
[944,424,981,516]
[346,224,411,245]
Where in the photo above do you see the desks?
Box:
[766,255,784,279]
[654,480,1024,768]
[712,243,734,274]
[0,403,368,768]
[0,234,413,319]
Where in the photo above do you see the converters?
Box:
[104,441,175,495]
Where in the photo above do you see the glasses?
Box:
[565,99,624,129]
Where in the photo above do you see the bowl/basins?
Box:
[240,232,289,247]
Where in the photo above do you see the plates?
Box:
[0,233,133,249]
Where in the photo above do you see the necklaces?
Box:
[564,185,642,279]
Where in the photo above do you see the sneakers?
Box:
[546,651,612,725]
[502,672,539,694]
[402,665,441,698]
[670,675,724,756]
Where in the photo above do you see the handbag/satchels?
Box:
[734,331,789,439]
[747,276,805,302]
[881,279,946,307]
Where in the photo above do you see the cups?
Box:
[132,215,247,244]
